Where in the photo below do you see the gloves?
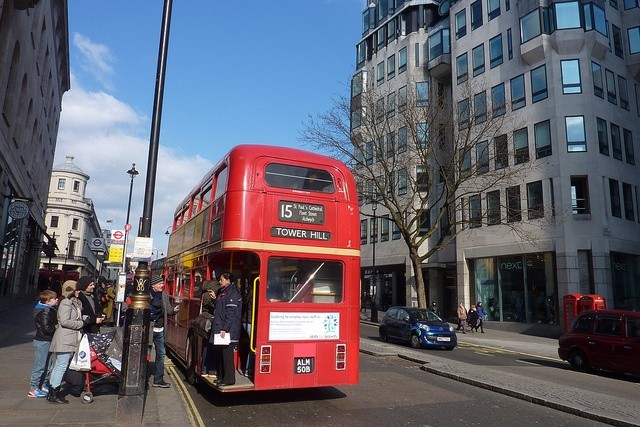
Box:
[83,316,91,325]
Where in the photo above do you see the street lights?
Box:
[152,249,163,259]
[371,193,378,322]
[116,163,139,326]
[64,230,72,280]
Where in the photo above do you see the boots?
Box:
[48,385,70,405]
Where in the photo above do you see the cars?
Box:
[379,306,457,350]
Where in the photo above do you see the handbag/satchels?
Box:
[192,311,215,340]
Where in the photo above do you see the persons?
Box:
[46,279,92,405]
[294,272,314,302]
[147,275,180,388]
[27,288,59,399]
[96,278,134,327]
[242,277,271,378]
[361,292,368,312]
[456,303,468,334]
[201,266,223,378]
[468,304,480,333]
[206,271,242,387]
[77,275,107,334]
[429,300,439,320]
[476,301,488,333]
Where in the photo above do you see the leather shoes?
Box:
[217,381,235,386]
[213,378,223,383]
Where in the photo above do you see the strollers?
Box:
[80,324,124,403]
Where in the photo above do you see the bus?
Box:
[163,144,361,393]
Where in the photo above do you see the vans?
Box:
[558,309,640,373]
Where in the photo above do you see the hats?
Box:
[79,275,93,290]
[151,274,163,283]
[60,280,78,299]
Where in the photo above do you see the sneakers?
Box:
[152,382,170,388]
[28,386,48,397]
[40,385,49,392]
